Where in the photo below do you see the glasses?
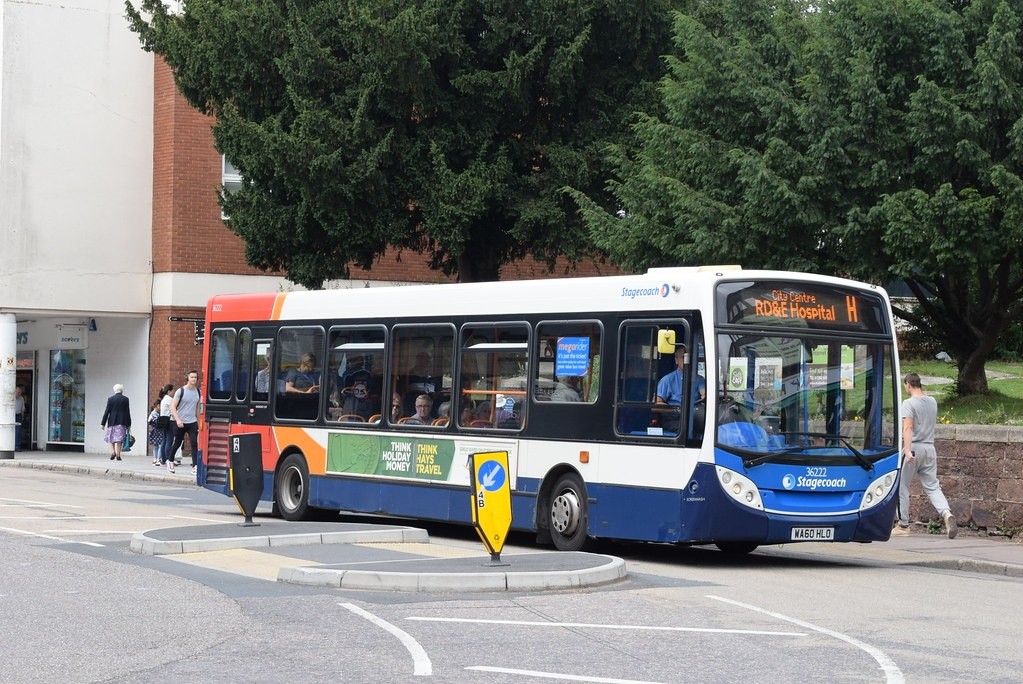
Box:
[415,405,432,409]
[481,409,491,413]
[392,404,400,409]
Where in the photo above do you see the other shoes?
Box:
[110,452,197,476]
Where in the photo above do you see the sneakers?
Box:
[890,524,910,537]
[944,513,958,540]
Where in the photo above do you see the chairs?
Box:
[210,353,674,438]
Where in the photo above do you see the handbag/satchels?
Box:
[156,416,171,427]
[128,427,135,447]
[121,428,131,451]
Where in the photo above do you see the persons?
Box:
[159,384,180,466]
[890,372,959,539]
[16,384,25,452]
[165,370,201,476]
[101,384,131,461]
[148,399,161,465]
[256,343,708,440]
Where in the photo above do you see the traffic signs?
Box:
[467,451,514,566]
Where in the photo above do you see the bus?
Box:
[196,264,902,557]
